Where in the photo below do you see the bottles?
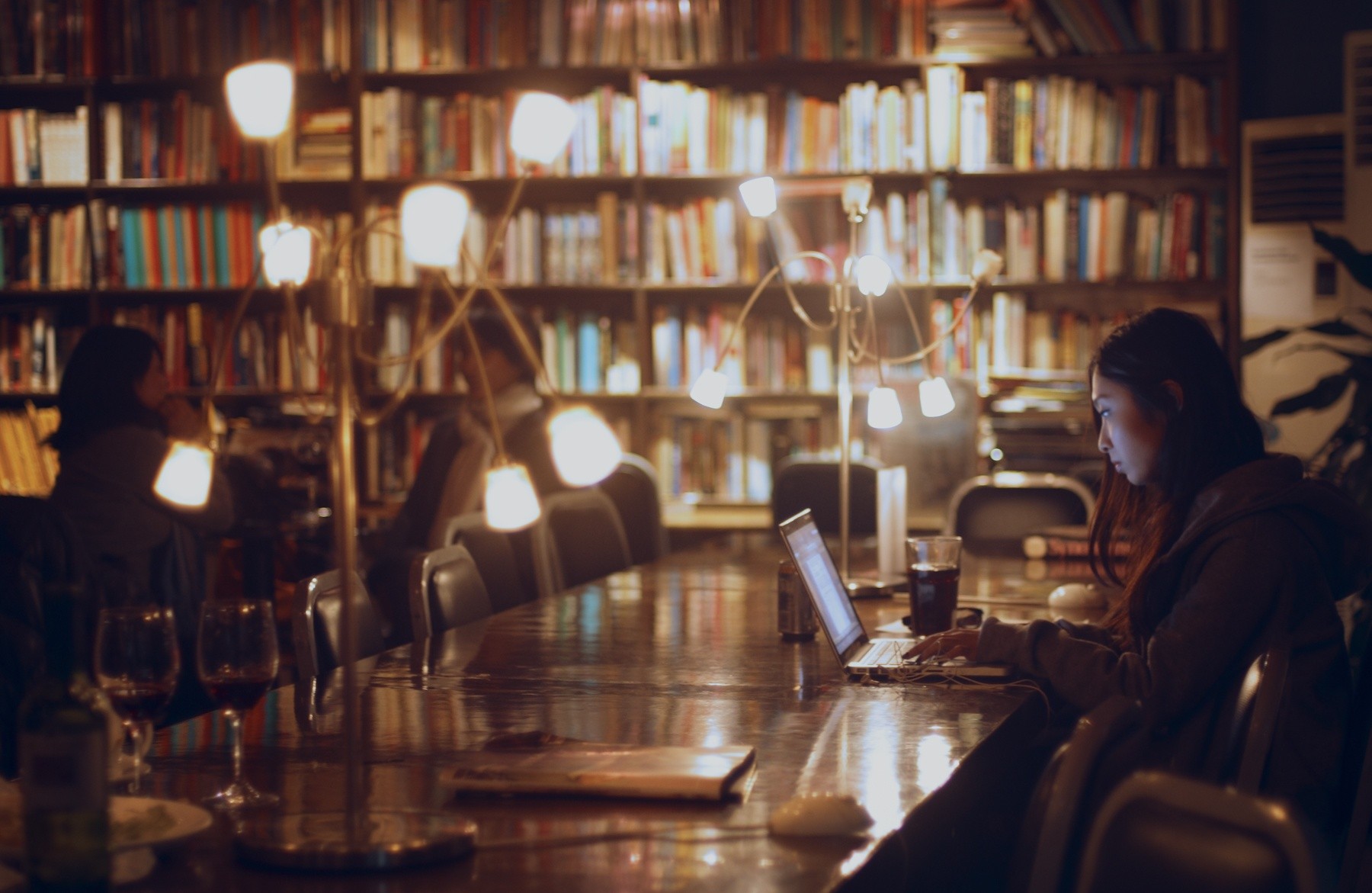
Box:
[12,556,113,893]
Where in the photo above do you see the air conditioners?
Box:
[1237,112,1342,336]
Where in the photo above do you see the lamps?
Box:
[688,178,1003,598]
[151,61,621,876]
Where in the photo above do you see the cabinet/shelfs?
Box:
[0,0,1236,532]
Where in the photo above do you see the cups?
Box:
[903,537,962,637]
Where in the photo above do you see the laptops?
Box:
[779,507,1011,681]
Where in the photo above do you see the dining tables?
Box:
[1,550,1133,893]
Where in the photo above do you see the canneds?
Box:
[779,560,819,637]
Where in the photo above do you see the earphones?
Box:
[859,673,881,686]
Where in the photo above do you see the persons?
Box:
[900,308,1372,892]
[367,289,554,647]
[36,325,233,656]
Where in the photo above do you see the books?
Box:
[438,729,756,801]
[0,0,1230,514]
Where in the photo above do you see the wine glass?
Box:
[89,600,183,803]
[194,601,283,814]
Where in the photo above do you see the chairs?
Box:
[1008,652,1334,893]
[946,471,1096,550]
[294,453,664,680]
